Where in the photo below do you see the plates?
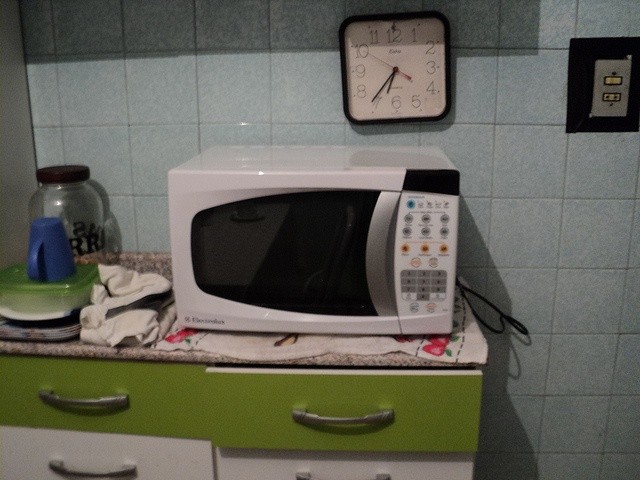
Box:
[0,323,82,342]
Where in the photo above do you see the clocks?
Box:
[339,11,452,125]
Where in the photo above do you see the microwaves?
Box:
[167,145,462,339]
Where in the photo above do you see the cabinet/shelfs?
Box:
[1,343,214,480]
[205,356,484,478]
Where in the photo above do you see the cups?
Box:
[27,216,77,282]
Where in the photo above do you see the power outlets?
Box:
[564,37,640,133]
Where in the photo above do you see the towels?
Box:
[153,292,490,365]
[78,262,178,348]
